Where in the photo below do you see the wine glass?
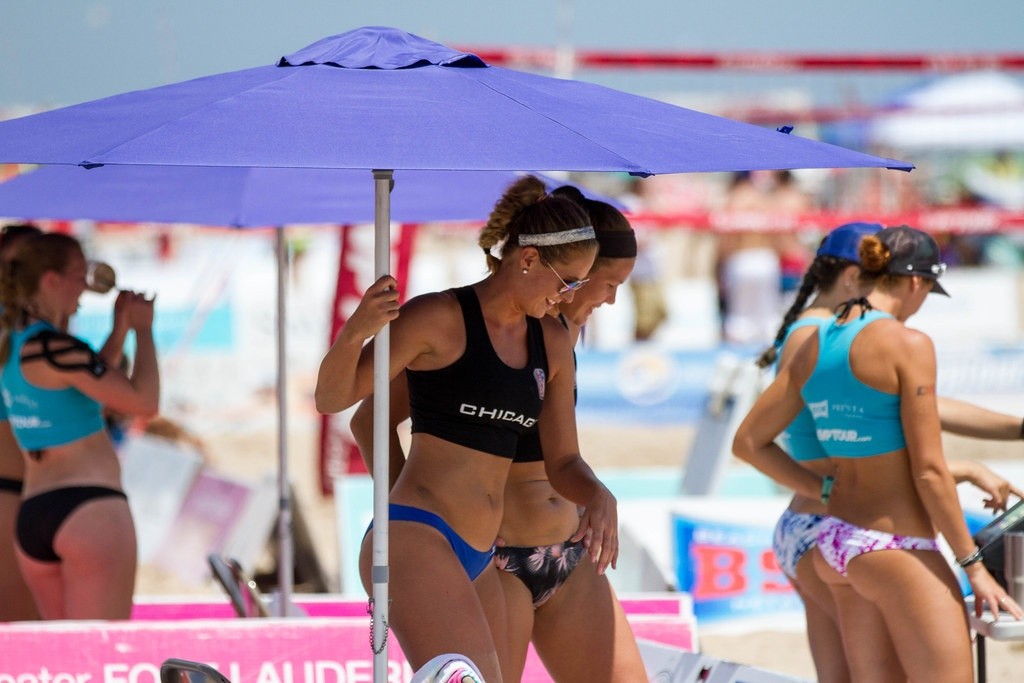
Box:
[85,260,122,295]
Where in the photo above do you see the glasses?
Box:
[546,261,591,294]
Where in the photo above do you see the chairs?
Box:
[209,554,273,618]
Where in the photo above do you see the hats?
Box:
[862,225,951,297]
[816,223,884,265]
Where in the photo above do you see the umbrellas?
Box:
[0,29,914,683]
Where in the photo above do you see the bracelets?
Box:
[820,474,835,505]
[956,546,984,568]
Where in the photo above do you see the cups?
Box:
[1003,533,1024,610]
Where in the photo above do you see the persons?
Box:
[731,223,1022,681]
[314,176,651,683]
[0,223,161,619]
